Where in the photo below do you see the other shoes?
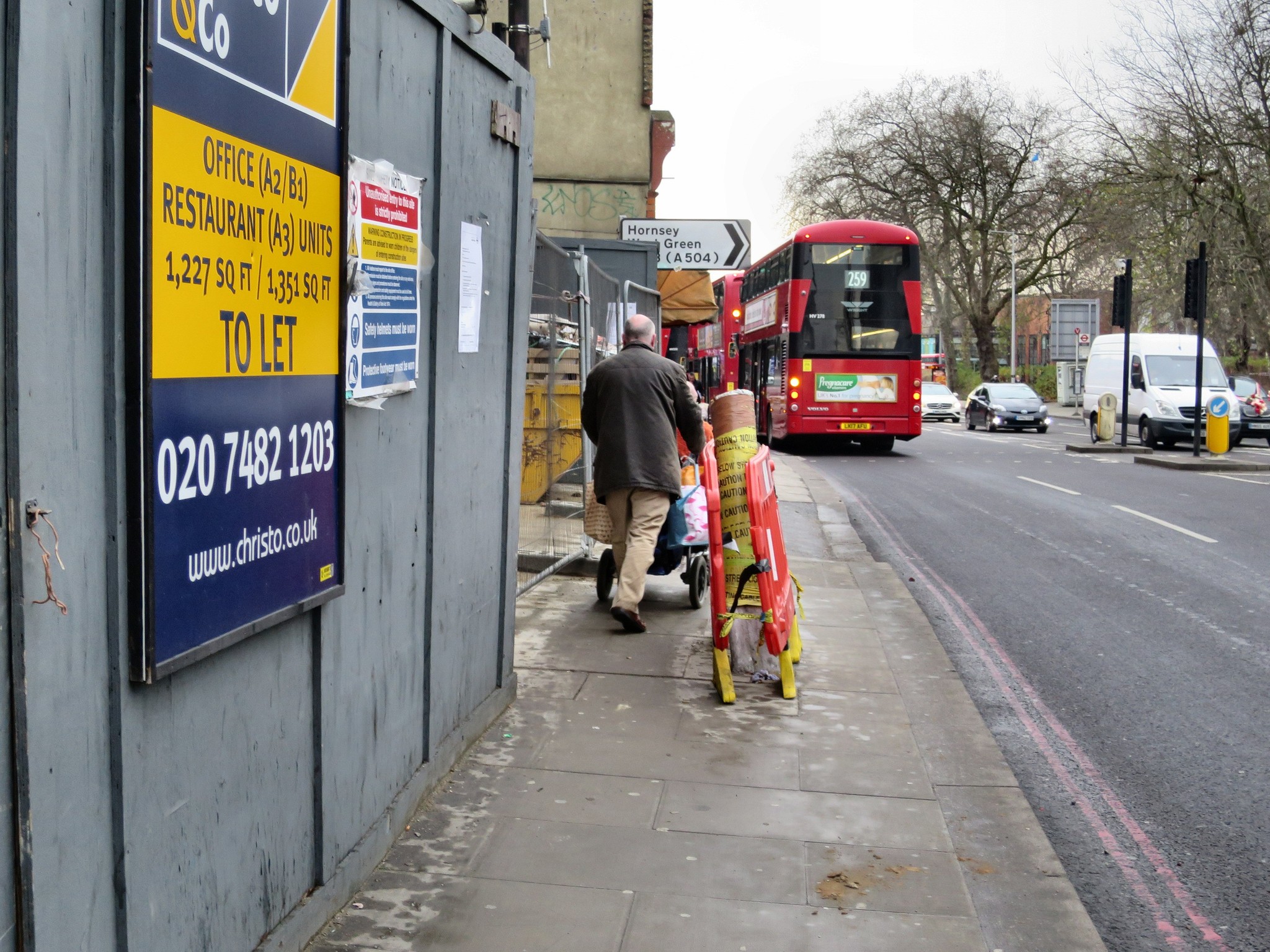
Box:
[609,607,646,631]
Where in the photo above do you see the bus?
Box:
[920,353,950,371]
[659,221,923,452]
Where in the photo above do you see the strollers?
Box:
[597,452,710,609]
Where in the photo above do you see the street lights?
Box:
[987,230,1016,383]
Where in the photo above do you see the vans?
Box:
[1082,333,1242,452]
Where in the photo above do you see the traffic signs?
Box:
[618,218,753,272]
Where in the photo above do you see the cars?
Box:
[965,382,1051,433]
[921,381,961,423]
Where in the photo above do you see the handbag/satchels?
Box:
[583,501,613,544]
[668,455,709,545]
[647,456,701,576]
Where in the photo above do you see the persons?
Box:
[581,314,713,634]
[990,375,1000,383]
[857,375,896,401]
[1015,375,1023,383]
[1140,373,1145,390]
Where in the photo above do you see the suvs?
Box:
[1225,374,1270,446]
